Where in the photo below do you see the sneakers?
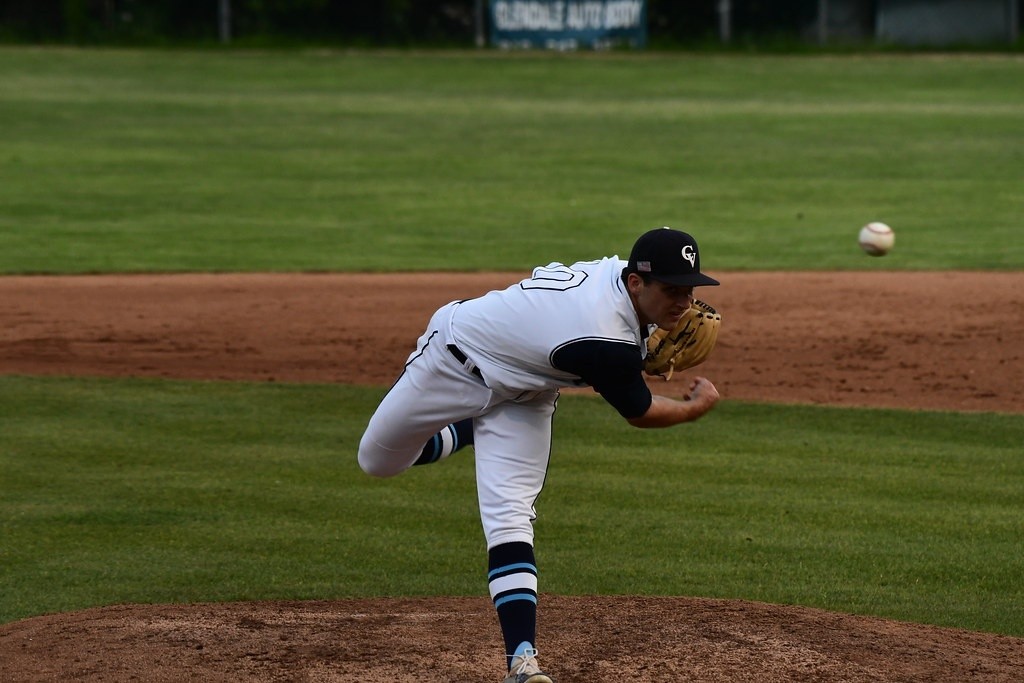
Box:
[504,648,553,683]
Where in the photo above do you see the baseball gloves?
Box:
[643,294,723,383]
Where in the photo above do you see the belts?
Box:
[447,345,484,380]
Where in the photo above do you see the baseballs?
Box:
[857,221,897,257]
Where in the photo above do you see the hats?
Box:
[628,227,720,287]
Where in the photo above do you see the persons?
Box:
[357,226,720,683]
